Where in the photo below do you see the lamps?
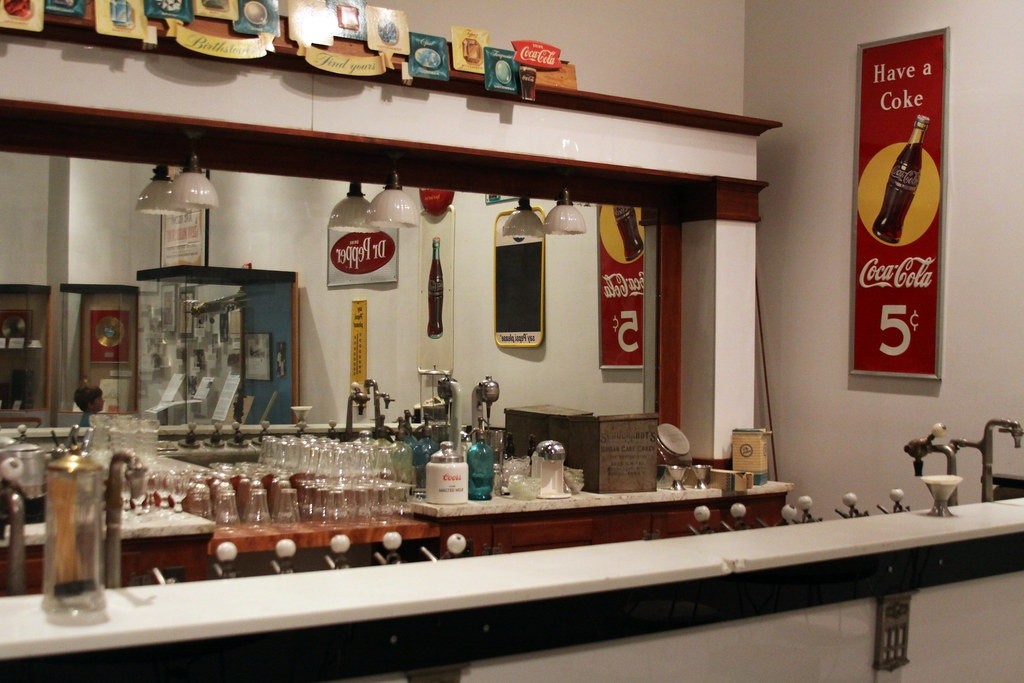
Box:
[135,165,188,216]
[544,188,586,235]
[171,153,219,210]
[501,197,544,238]
[370,170,419,229]
[327,181,380,234]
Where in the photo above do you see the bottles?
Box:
[873,114,930,244]
[426,441,470,504]
[427,236,444,338]
[41,443,107,620]
[373,409,436,490]
[466,417,494,501]
[613,205,644,261]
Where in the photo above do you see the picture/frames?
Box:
[161,283,177,333]
[243,330,273,382]
[178,291,195,339]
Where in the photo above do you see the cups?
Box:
[921,474,962,518]
[86,413,416,525]
[665,464,713,491]
[519,66,537,102]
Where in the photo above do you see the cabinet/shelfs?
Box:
[414,492,787,559]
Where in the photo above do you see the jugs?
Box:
[0,424,96,524]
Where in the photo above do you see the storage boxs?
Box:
[710,468,754,492]
